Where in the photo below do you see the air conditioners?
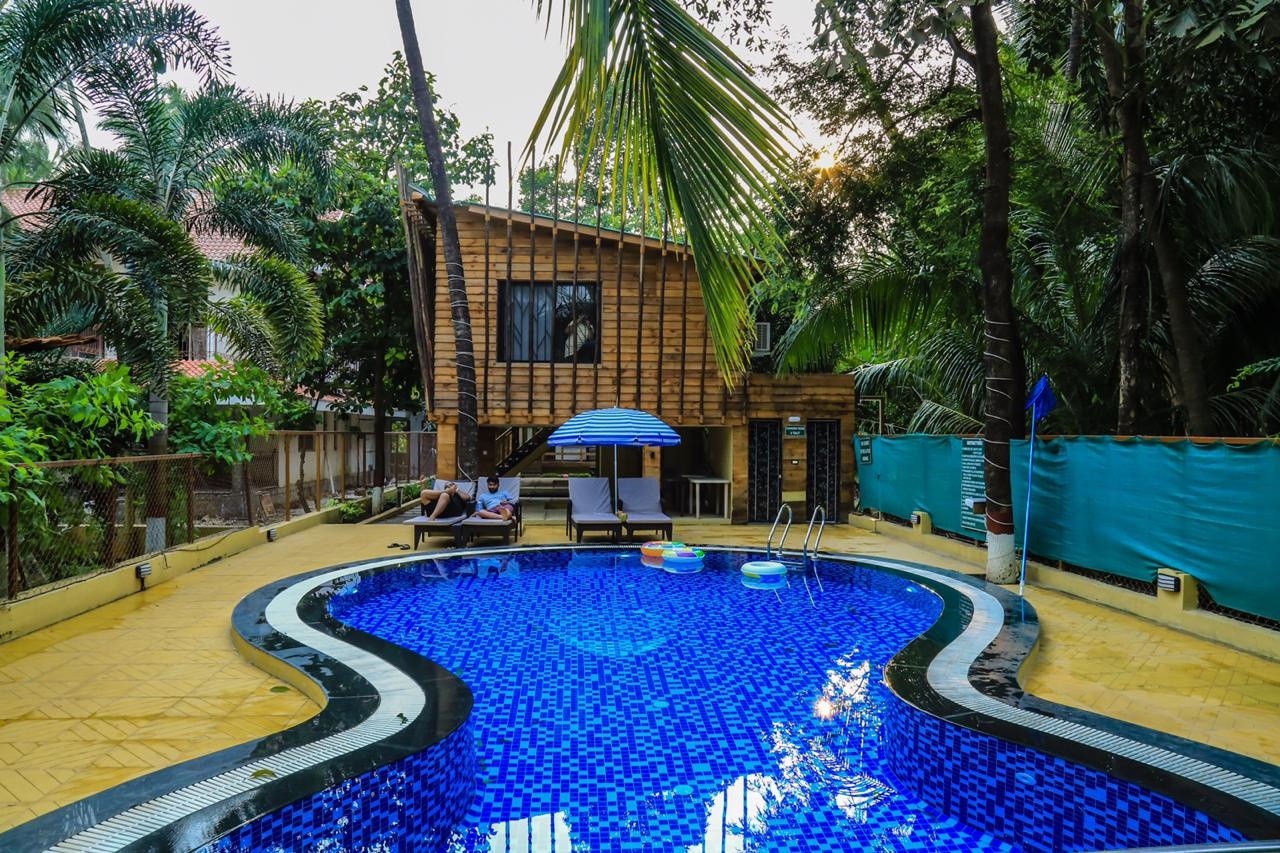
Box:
[753,321,771,353]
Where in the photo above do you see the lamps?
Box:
[1157,572,1181,593]
[909,513,921,525]
[135,562,153,590]
[266,527,278,544]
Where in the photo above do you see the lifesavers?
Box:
[640,540,687,558]
[740,561,788,579]
[640,555,665,568]
[661,547,706,563]
[663,560,705,574]
[741,576,788,590]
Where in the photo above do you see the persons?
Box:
[471,475,516,522]
[420,481,472,521]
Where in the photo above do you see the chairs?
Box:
[565,475,623,545]
[616,475,674,542]
[461,476,523,548]
[402,478,477,551]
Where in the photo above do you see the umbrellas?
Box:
[547,405,682,517]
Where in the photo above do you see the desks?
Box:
[687,476,731,520]
